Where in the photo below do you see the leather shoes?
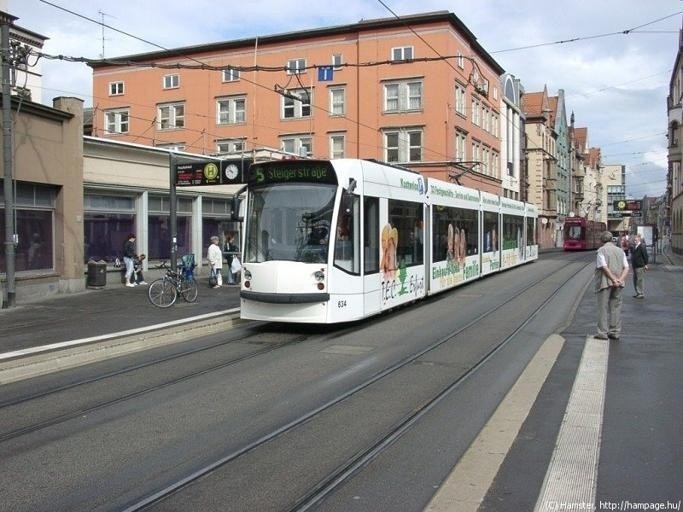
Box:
[608,335,619,340]
[594,335,608,340]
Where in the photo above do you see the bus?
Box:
[561,200,610,251]
[234,156,540,327]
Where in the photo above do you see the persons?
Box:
[122,233,136,287]
[459,229,465,268]
[134,253,146,285]
[224,233,239,285]
[379,224,391,269]
[454,227,460,261]
[206,235,223,289]
[491,227,498,255]
[385,227,398,274]
[629,234,648,299]
[612,231,629,256]
[590,229,629,341]
[445,224,453,261]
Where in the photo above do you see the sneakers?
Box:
[125,282,134,288]
[138,281,147,285]
[635,294,643,298]
[132,282,137,285]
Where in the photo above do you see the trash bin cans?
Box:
[87,256,107,286]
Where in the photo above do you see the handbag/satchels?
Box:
[208,269,216,286]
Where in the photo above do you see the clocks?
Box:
[223,162,240,182]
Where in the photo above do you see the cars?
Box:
[612,235,628,242]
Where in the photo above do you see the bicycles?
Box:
[145,260,199,310]
